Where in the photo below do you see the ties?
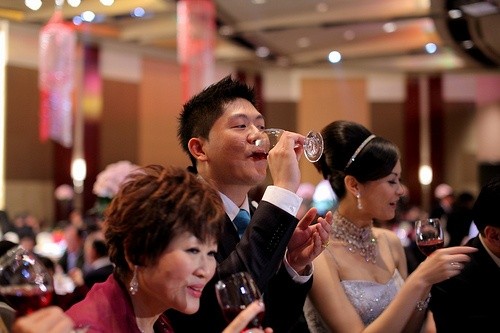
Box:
[233,209,251,240]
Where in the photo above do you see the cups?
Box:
[215,272,266,332]
[415,218,445,257]
[0,245,54,316]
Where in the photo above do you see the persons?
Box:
[372,160,500,333]
[153,73,334,333]
[307,120,478,333]
[0,161,273,333]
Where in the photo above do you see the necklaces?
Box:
[331,207,378,264]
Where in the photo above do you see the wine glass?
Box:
[256,128,324,163]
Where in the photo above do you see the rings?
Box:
[321,241,330,248]
[450,261,460,270]
